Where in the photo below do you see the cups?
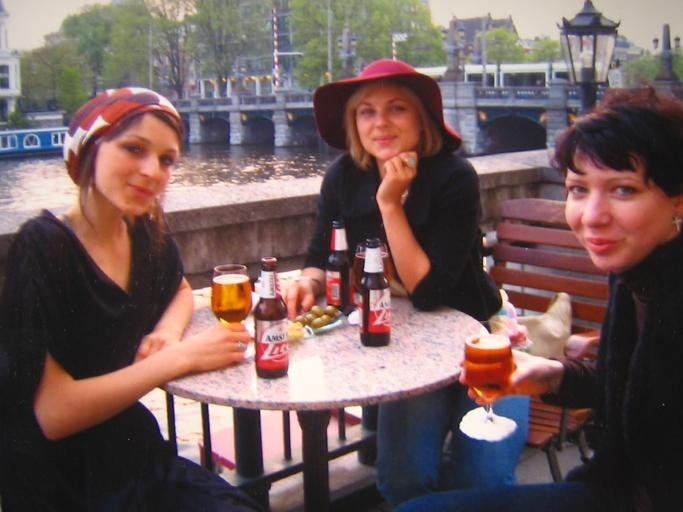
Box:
[351,239,393,327]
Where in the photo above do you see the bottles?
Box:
[254,256,290,379]
[325,219,354,315]
[360,236,392,347]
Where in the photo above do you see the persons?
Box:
[387,79,683,512]
[0,84,271,511]
[278,57,531,510]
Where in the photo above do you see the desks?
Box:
[156,280,490,512]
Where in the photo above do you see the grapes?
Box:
[294,305,336,329]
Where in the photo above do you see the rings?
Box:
[402,158,416,167]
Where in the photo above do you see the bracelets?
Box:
[293,277,323,296]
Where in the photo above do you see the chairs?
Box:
[486,196,608,482]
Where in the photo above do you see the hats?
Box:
[63,87,181,189]
[313,59,462,152]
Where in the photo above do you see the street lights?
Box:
[555,0,621,117]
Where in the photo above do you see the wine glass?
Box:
[211,264,252,365]
[460,320,518,442]
